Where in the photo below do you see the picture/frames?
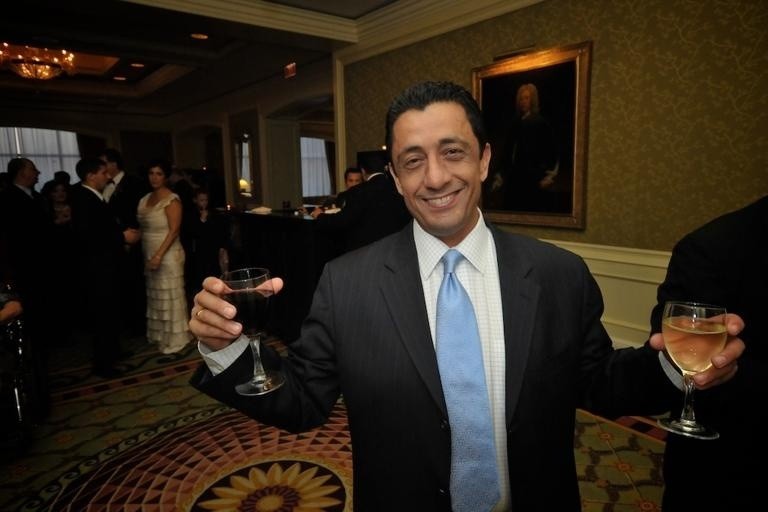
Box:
[469,39,589,232]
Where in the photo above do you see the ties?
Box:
[436,249,501,512]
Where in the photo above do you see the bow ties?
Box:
[105,179,115,185]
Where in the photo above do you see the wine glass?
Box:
[219,267,288,397]
[656,300,729,440]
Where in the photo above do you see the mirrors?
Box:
[227,91,336,212]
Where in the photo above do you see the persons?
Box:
[1,150,220,431]
[490,83,563,210]
[297,167,367,214]
[311,154,413,260]
[184,80,749,512]
[646,194,768,511]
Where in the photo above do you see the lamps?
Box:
[0,40,81,83]
